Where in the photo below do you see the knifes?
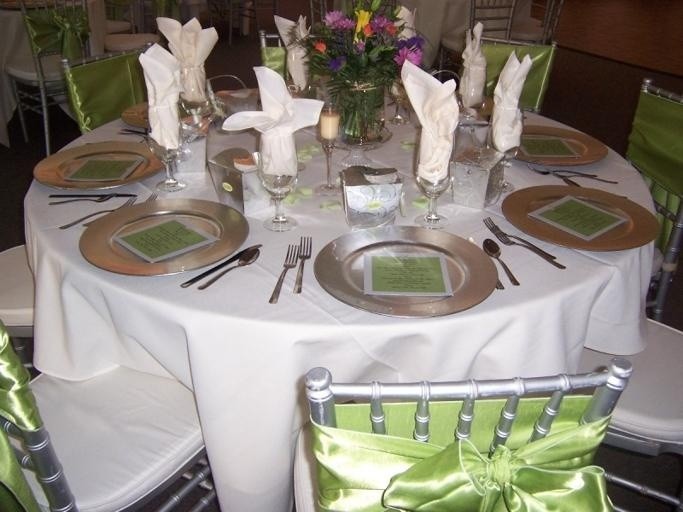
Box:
[181,245,262,290]
[49,191,137,197]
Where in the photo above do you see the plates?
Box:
[501,184,662,251]
[33,141,162,191]
[313,226,498,317]
[120,101,150,130]
[77,198,250,276]
[512,125,608,165]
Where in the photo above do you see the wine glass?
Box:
[388,78,411,125]
[283,51,314,98]
[316,103,342,195]
[487,119,520,191]
[179,79,211,138]
[147,104,189,191]
[458,78,482,133]
[412,138,454,229]
[257,140,300,232]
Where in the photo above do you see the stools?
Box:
[106,33,160,54]
[106,19,130,33]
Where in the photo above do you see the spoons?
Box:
[196,248,260,291]
[482,237,522,287]
[46,193,117,205]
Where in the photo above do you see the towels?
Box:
[139,42,186,150]
[397,4,417,40]
[458,22,486,106]
[222,66,325,176]
[492,49,531,153]
[400,58,459,184]
[274,13,313,90]
[156,16,218,103]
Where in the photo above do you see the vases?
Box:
[338,80,393,152]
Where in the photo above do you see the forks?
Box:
[293,235,314,293]
[546,165,618,189]
[482,217,557,259]
[58,197,138,244]
[83,192,159,231]
[526,163,598,181]
[493,229,566,270]
[267,244,298,305]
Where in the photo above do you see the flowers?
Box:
[283,0,432,144]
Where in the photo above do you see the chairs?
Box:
[1,311,226,512]
[258,28,297,85]
[507,0,562,49]
[62,43,151,137]
[436,3,514,90]
[623,75,681,320]
[481,36,559,114]
[291,356,633,512]
[3,1,98,158]
[207,0,261,49]
[2,240,40,364]
[573,315,683,509]
